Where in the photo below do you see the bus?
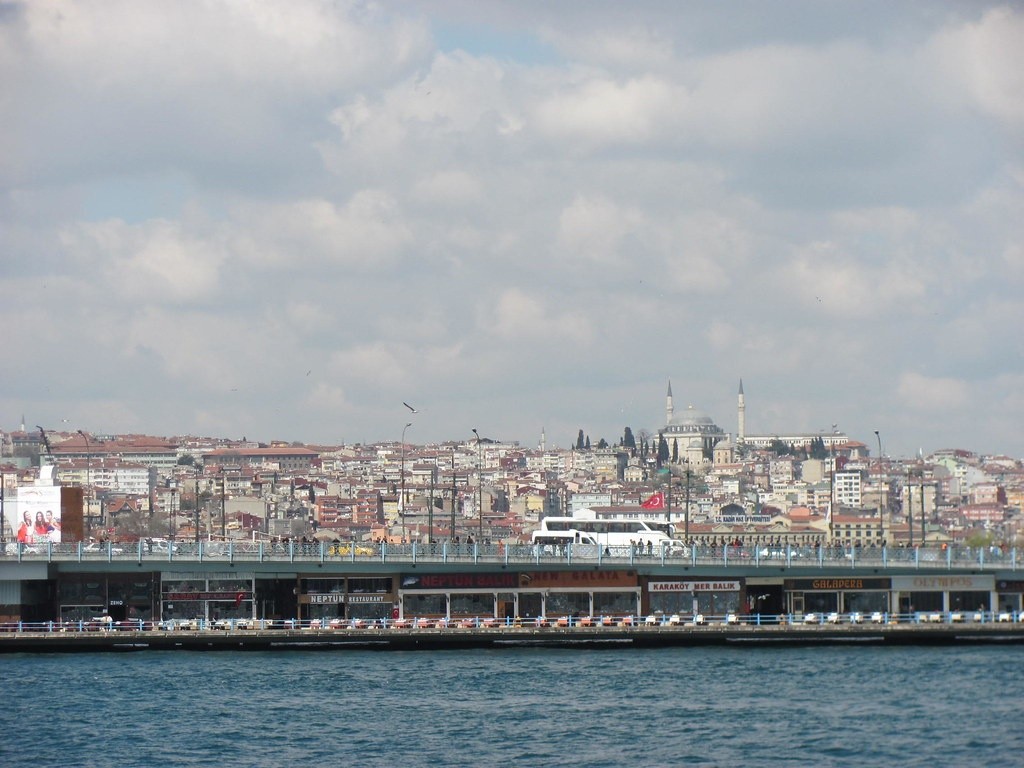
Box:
[541,516,677,559]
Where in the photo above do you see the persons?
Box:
[711,538,811,560]
[666,540,707,559]
[942,542,1021,563]
[271,537,394,556]
[19,511,61,550]
[519,538,609,560]
[99,536,225,556]
[815,540,916,561]
[374,611,381,629]
[630,538,653,558]
[0,618,216,631]
[400,536,504,560]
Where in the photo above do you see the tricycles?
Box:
[660,539,691,560]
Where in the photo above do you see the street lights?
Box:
[401,421,413,546]
[829,422,838,545]
[76,428,91,541]
[874,429,882,545]
[470,427,482,543]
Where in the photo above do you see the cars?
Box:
[81,543,123,556]
[757,547,804,559]
[328,540,376,559]
[4,542,41,557]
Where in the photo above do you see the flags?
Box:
[642,492,664,509]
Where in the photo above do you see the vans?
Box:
[143,538,179,556]
[531,530,599,559]
